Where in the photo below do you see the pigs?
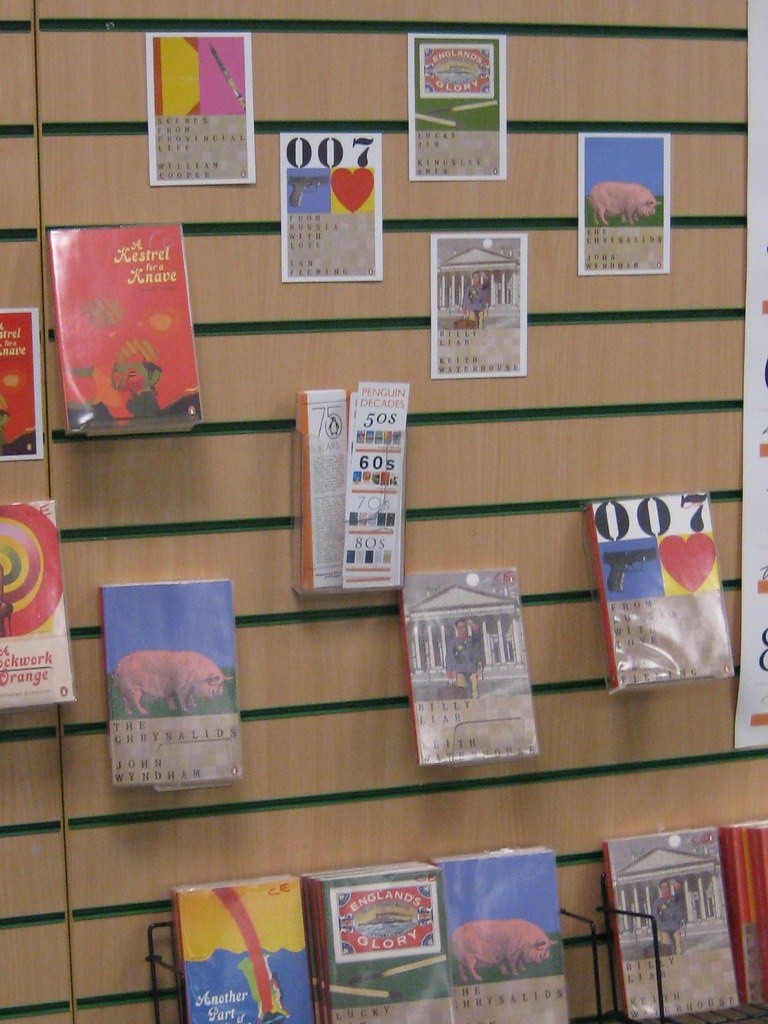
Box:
[448,919,557,981]
[112,650,233,715]
[588,182,658,225]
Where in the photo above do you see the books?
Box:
[48,224,205,434]
[396,566,540,766]
[0,499,76,710]
[99,578,245,789]
[169,845,568,1023]
[602,819,768,1020]
[584,490,736,694]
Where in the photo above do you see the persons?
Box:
[464,273,489,328]
[446,620,484,700]
[651,879,687,956]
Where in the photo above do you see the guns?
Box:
[605,548,656,592]
